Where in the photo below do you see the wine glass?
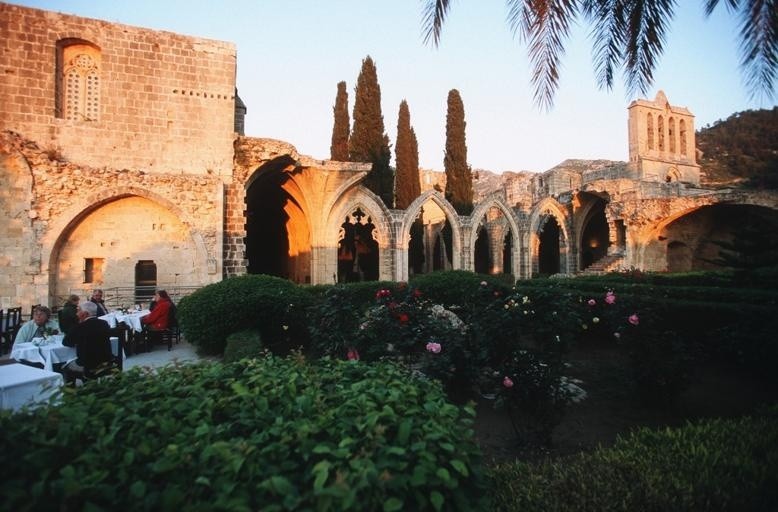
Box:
[135,304,140,315]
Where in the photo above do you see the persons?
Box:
[59,295,82,334]
[10,305,62,372]
[148,294,180,329]
[89,288,108,319]
[138,289,171,333]
[63,301,114,387]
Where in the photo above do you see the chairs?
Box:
[0,303,180,387]
